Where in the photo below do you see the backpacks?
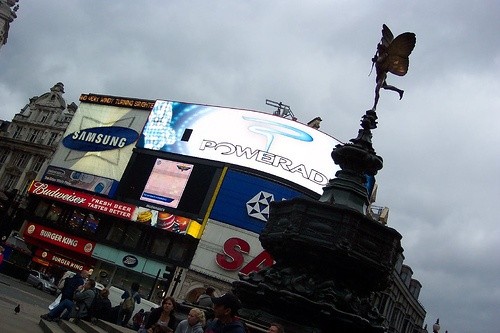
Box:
[58,276,70,289]
[122,289,139,313]
[87,289,105,319]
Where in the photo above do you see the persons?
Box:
[196,287,215,308]
[269,323,283,333]
[93,288,111,321]
[117,282,141,326]
[145,296,178,333]
[0,246,4,264]
[132,306,155,332]
[372,43,404,111]
[40,279,96,322]
[60,270,89,304]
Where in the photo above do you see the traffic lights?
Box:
[160,266,176,290]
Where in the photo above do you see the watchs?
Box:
[176,308,204,333]
[205,292,246,333]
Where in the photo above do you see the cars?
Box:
[27,270,58,295]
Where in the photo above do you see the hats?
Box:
[214,293,241,306]
